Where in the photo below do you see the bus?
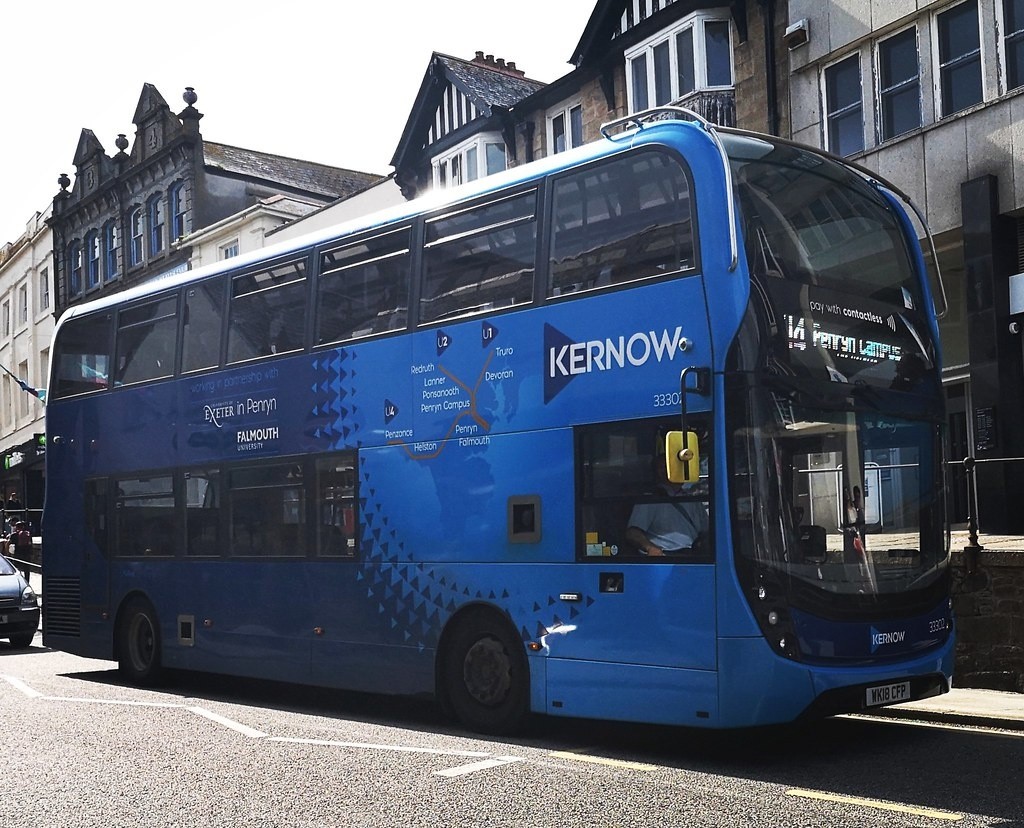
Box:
[40,106,956,736]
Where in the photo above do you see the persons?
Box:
[626,453,710,558]
[1,493,31,582]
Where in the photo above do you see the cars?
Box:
[0,553,40,648]
